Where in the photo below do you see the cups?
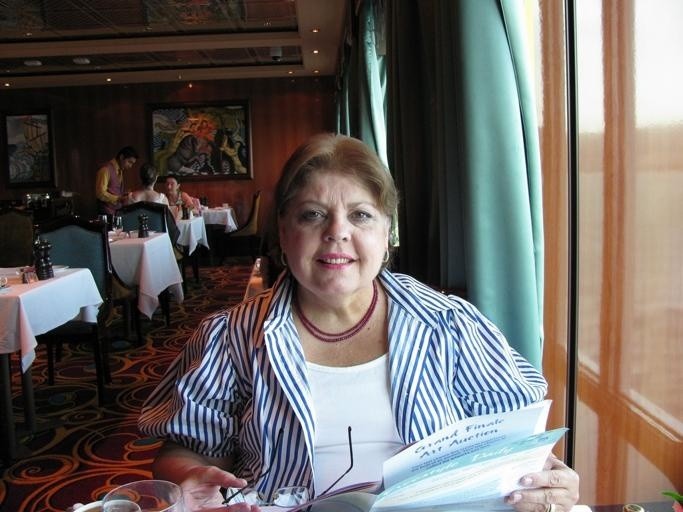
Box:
[98,214,107,224]
[70,479,185,512]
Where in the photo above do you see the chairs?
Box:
[218,189,262,264]
[111,200,168,234]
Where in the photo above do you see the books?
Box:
[288,400,568,512]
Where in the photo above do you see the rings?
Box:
[547,503,556,512]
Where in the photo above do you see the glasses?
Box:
[222,426,353,507]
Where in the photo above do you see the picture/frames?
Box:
[2,107,58,191]
[143,97,254,182]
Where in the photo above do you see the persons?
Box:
[95,145,139,222]
[135,130,581,512]
[165,174,198,215]
[126,161,169,206]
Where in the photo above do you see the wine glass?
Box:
[112,216,124,240]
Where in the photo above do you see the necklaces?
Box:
[293,280,378,343]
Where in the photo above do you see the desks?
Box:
[0,205,232,460]
